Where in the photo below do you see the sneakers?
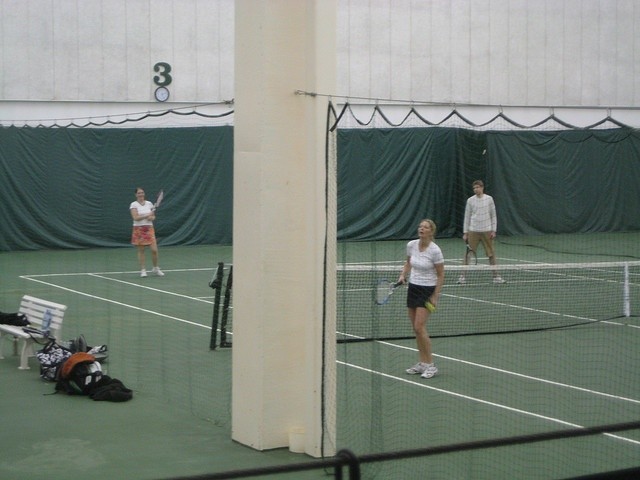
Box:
[152,266,164,277]
[141,268,148,277]
[493,274,508,285]
[457,275,467,284]
[406,361,426,374]
[421,362,439,379]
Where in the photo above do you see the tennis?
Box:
[425,302,435,313]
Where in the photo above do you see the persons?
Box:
[456,180,507,284]
[398,218,445,379]
[129,187,164,277]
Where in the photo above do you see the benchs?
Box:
[0,295,67,370]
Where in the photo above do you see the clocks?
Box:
[154,84,170,102]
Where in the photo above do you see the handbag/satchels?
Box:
[35,341,71,380]
[86,372,132,401]
